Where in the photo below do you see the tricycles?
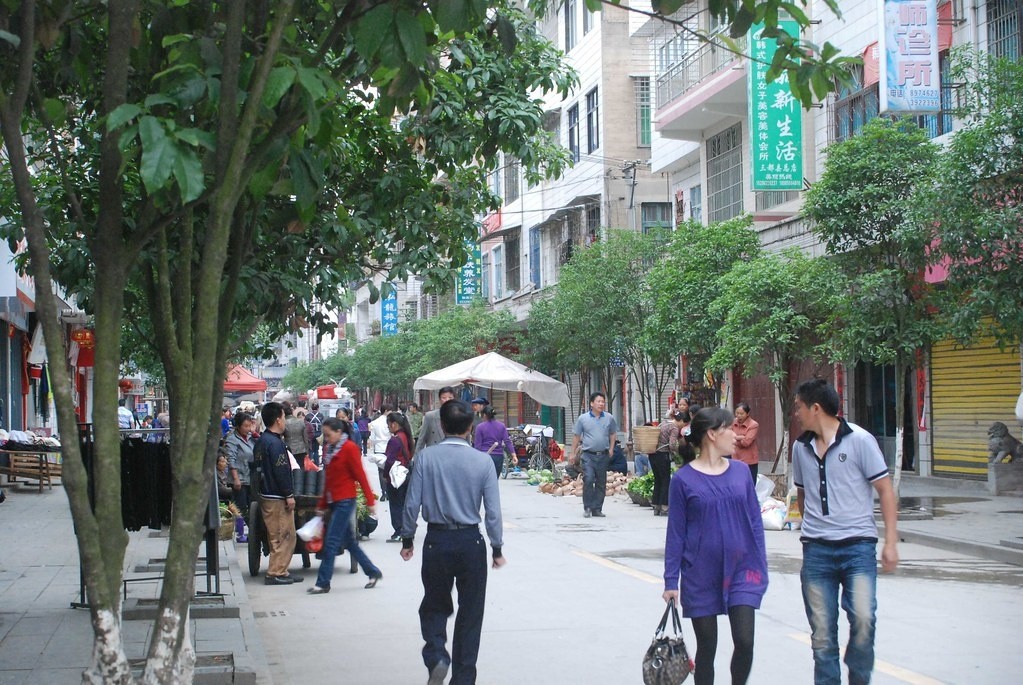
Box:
[501,424,555,480]
[247,491,359,577]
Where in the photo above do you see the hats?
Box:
[471,398,486,404]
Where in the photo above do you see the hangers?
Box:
[120,428,171,445]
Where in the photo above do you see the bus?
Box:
[306,385,356,423]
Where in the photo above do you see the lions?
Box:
[987,422,1023,464]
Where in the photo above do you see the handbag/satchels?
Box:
[642,599,691,685]
[305,524,326,552]
[296,516,324,541]
[406,460,414,474]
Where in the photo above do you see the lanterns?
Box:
[121,379,133,390]
[72,329,94,349]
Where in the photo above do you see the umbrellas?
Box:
[413,351,569,408]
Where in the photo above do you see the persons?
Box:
[472,398,518,478]
[635,398,700,516]
[254,402,304,584]
[565,446,583,481]
[308,417,382,594]
[608,440,627,476]
[354,401,423,501]
[570,392,617,517]
[386,412,415,542]
[731,403,760,486]
[400,398,506,685]
[217,407,265,544]
[118,399,165,442]
[416,386,471,450]
[663,408,768,685]
[282,400,361,467]
[792,380,899,685]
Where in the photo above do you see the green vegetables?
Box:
[356,486,379,522]
[628,441,699,497]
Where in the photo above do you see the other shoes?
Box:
[365,573,382,588]
[427,658,448,685]
[279,573,304,581]
[583,507,591,517]
[654,504,669,516]
[592,509,605,517]
[307,586,330,593]
[265,575,294,584]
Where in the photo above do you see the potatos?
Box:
[554,470,630,497]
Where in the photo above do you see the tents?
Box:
[223,364,266,402]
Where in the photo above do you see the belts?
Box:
[428,523,478,530]
[583,450,609,456]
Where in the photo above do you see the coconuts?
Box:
[528,468,554,486]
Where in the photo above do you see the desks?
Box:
[1,449,63,494]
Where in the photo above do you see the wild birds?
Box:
[330,377,346,387]
